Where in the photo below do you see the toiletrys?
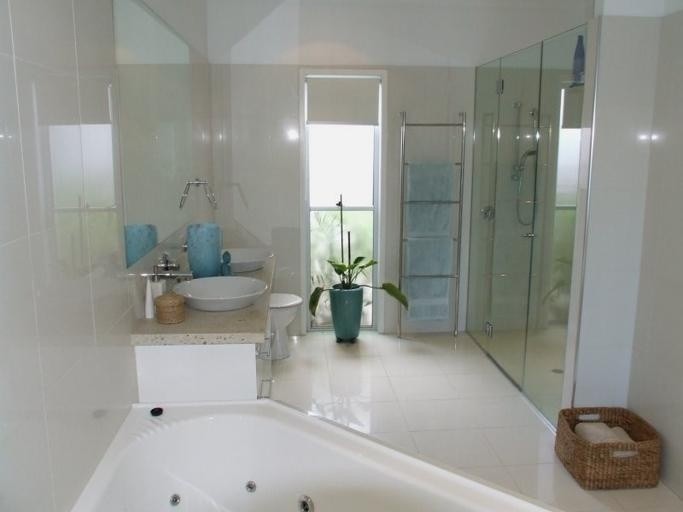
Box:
[144,277,155,318]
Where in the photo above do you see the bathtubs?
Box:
[74,398,565,510]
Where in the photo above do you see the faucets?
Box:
[152,250,193,282]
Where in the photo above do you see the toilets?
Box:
[270,293,303,360]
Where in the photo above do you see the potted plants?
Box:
[305,255,410,345]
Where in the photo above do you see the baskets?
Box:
[554,404,665,492]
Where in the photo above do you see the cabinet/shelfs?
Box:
[132,306,275,403]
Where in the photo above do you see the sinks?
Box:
[223,246,276,274]
[172,275,269,313]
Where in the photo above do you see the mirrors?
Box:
[110,1,211,274]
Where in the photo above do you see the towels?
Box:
[405,235,453,323]
[404,159,454,235]
[573,420,639,458]
